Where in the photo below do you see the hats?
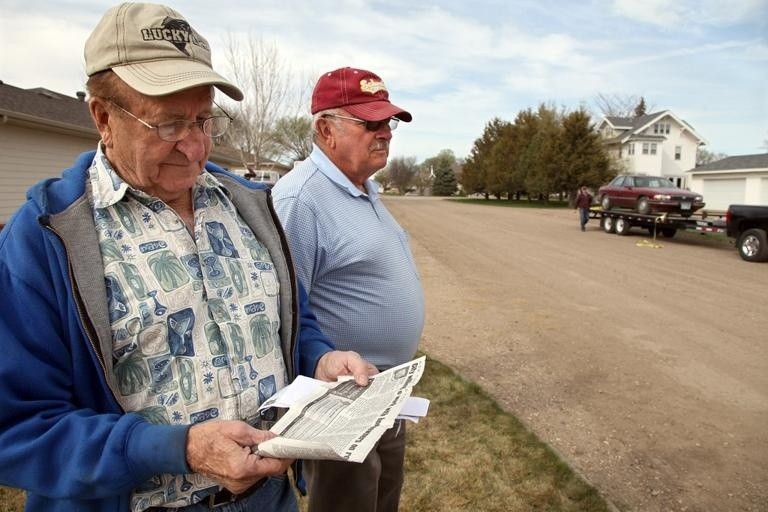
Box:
[311,66,411,122]
[83,2,245,102]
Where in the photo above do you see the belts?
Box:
[201,477,268,510]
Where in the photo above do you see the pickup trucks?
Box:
[725,204,767,262]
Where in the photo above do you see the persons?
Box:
[273,65,427,512]
[0,1,379,507]
[573,185,595,232]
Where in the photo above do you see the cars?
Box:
[596,174,705,217]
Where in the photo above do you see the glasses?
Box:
[107,100,233,142]
[333,115,399,132]
